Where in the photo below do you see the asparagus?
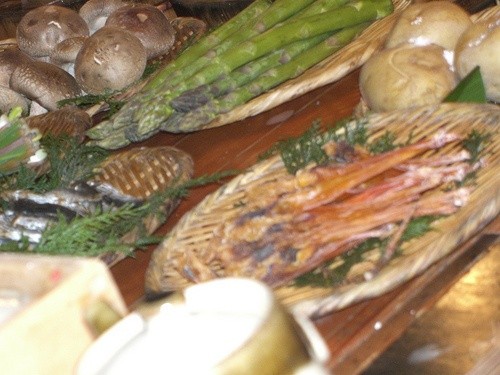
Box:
[83,0,395,151]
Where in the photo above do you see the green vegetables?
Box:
[0,106,239,258]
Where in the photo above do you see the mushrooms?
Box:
[359,0,500,112]
[0,0,175,125]
